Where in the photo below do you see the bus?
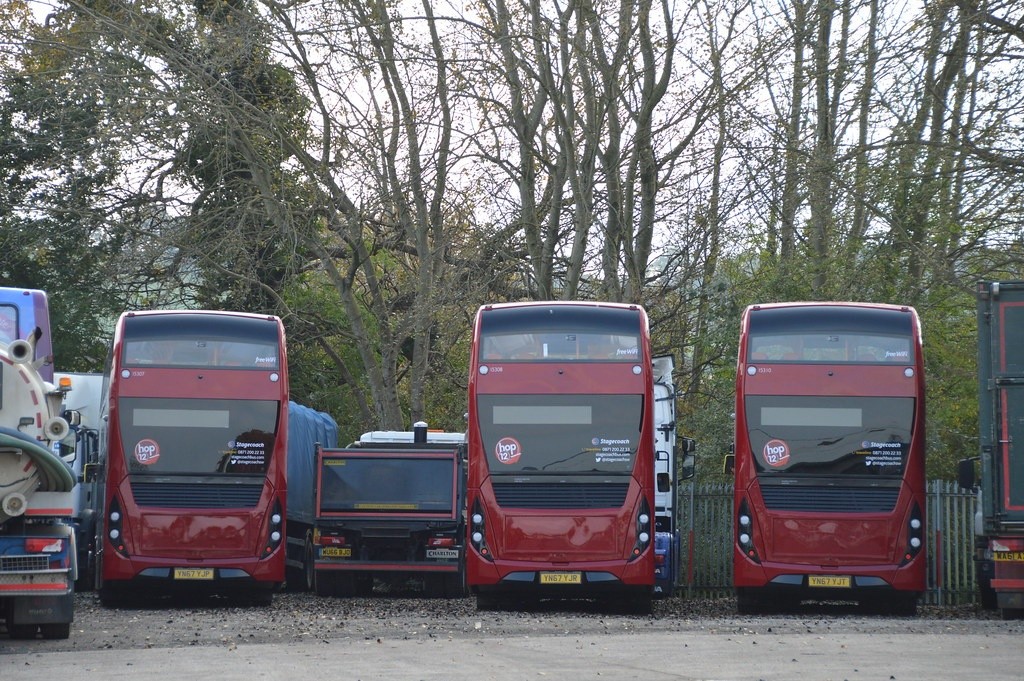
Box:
[647,355,697,595]
[723,300,933,616]
[956,280,1024,615]
[98,309,341,604]
[456,301,672,595]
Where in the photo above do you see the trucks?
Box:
[304,420,469,591]
[0,287,106,639]
